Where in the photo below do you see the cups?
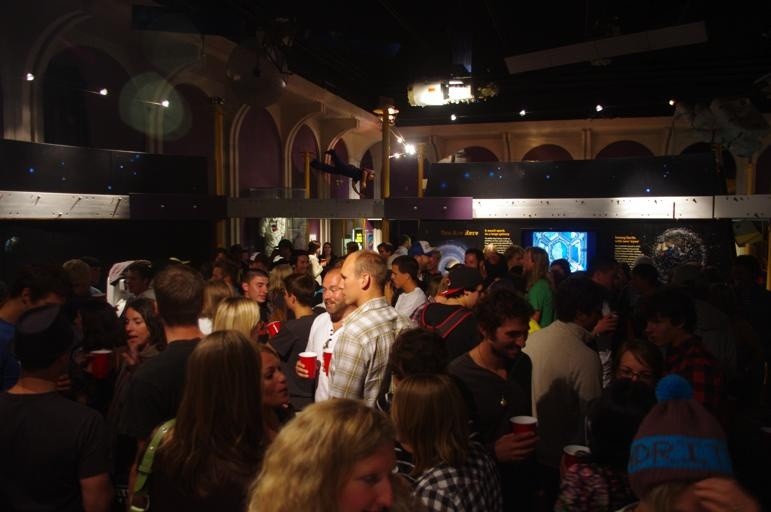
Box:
[90,349,111,379]
[562,445,590,473]
[509,416,537,457]
[265,320,281,338]
[321,258,326,265]
[323,351,331,377]
[297,352,317,380]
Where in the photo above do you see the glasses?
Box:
[615,368,654,381]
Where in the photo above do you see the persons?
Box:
[309,146,377,199]
[1,233,771,512]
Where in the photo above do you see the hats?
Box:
[627,373,733,495]
[14,303,73,362]
[440,265,483,295]
[409,241,435,256]
